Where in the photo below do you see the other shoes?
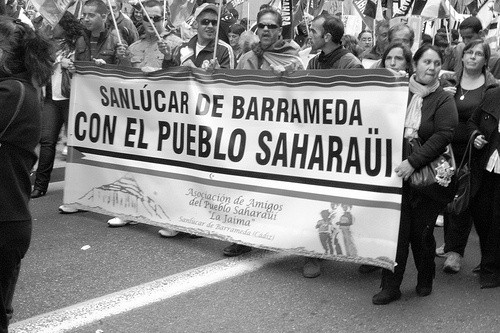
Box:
[372,286,400,304]
[159,229,179,237]
[416,271,431,296]
[223,243,250,256]
[59,205,79,213]
[303,255,320,277]
[107,217,132,227]
[474,264,481,271]
[359,263,377,272]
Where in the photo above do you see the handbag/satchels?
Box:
[408,137,452,199]
[60,67,72,98]
[441,129,482,214]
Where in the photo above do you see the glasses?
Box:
[463,49,486,58]
[257,23,280,29]
[142,15,163,22]
[197,19,218,26]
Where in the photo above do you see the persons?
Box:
[58,0,128,213]
[372,43,458,307]
[0,16,57,333]
[465,88,500,290]
[305,11,365,277]
[107,0,188,226]
[222,9,302,258]
[435,38,500,269]
[158,3,236,238]
[0,0,500,226]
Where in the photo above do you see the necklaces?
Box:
[459,83,472,101]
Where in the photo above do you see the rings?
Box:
[477,143,478,147]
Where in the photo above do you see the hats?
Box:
[194,2,219,20]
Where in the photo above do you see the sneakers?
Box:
[444,253,462,272]
[435,243,446,256]
[436,215,444,226]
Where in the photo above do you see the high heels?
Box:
[30,188,46,197]
